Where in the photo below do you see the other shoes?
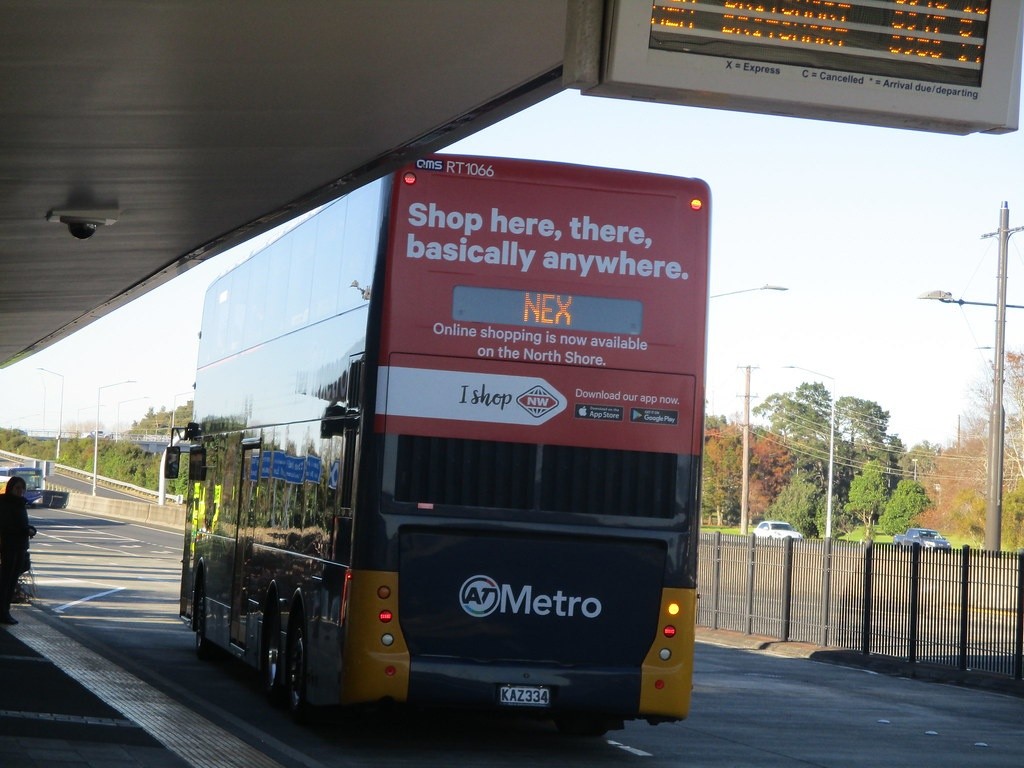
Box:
[0,616,18,625]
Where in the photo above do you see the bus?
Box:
[164,153,713,743]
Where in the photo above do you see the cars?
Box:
[752,520,803,539]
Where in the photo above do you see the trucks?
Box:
[0,467,70,509]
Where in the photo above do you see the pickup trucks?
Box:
[893,528,951,549]
[81,431,110,440]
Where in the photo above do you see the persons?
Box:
[0,476,37,625]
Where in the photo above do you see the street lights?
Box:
[116,396,150,443]
[36,367,65,463]
[785,365,837,539]
[917,201,1024,550]
[92,379,139,496]
